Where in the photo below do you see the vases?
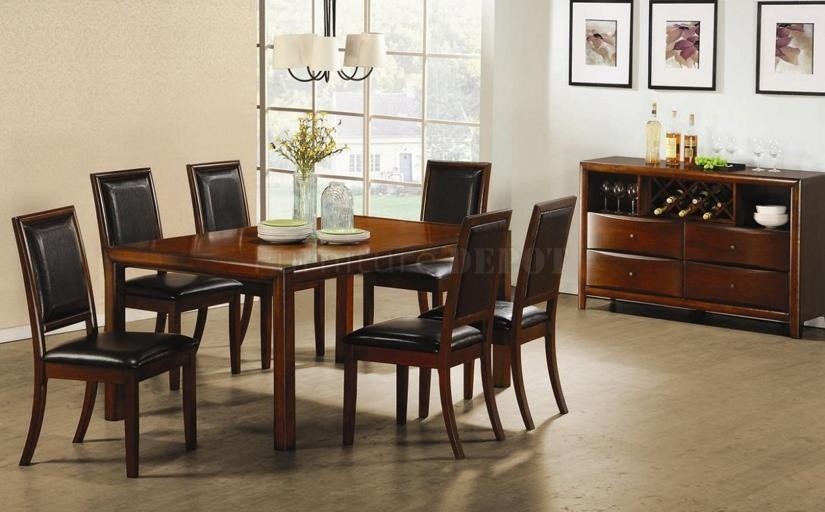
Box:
[292,171,319,233]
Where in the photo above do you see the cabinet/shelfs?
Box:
[573,154,825,343]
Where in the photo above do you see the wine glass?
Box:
[709,131,783,174]
[600,180,637,216]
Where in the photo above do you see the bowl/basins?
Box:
[756,204,786,215]
[755,212,789,229]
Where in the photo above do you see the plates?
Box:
[257,218,312,244]
[318,230,370,244]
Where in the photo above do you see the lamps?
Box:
[271,0,387,86]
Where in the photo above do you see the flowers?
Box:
[269,110,352,171]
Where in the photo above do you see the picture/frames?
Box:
[753,1,824,98]
[646,0,720,94]
[568,0,635,90]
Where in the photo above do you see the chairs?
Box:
[186,158,327,375]
[88,163,246,391]
[333,205,514,462]
[418,191,584,435]
[361,155,493,327]
[10,203,201,484]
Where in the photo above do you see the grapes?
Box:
[695,156,727,170]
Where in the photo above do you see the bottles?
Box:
[665,110,683,168]
[646,103,661,165]
[321,182,354,232]
[654,190,724,220]
[684,115,697,166]
